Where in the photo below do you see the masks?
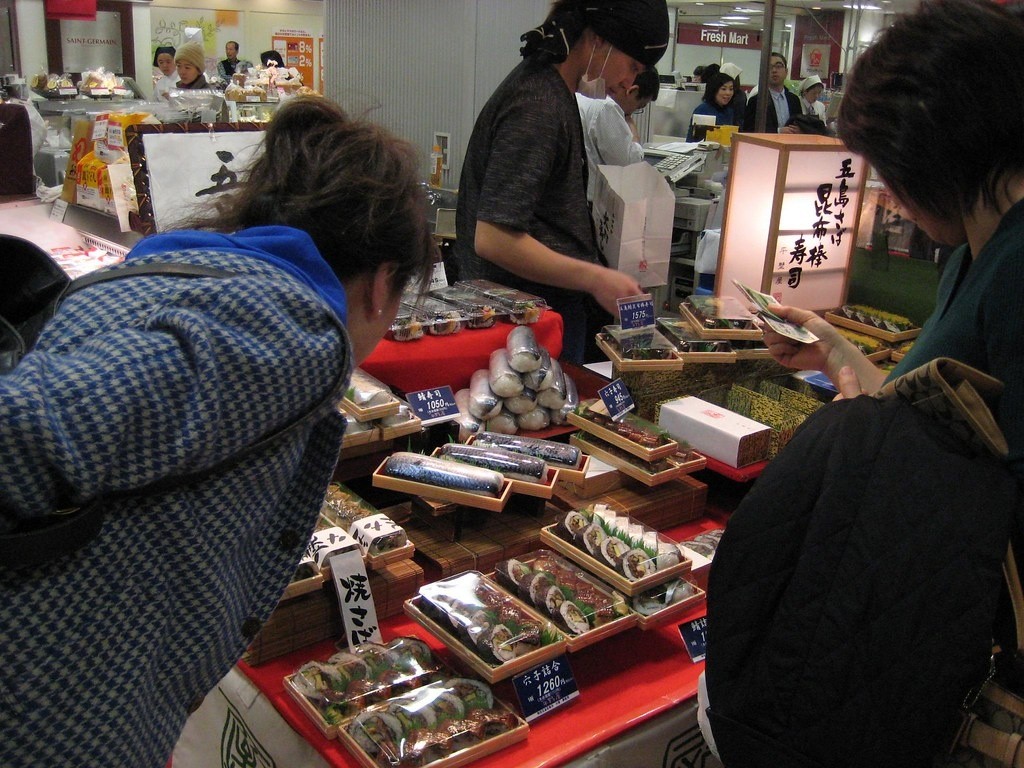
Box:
[576,42,613,99]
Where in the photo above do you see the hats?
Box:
[153,46,176,67]
[173,41,206,74]
[799,75,822,91]
[584,0,670,68]
[719,63,742,80]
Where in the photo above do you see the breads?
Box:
[224,72,324,102]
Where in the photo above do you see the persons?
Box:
[0,96,439,768]
[453,0,669,369]
[217,41,253,94]
[688,0,1024,768]
[260,50,304,86]
[174,42,211,90]
[153,47,181,97]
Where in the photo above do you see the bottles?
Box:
[430,145,443,187]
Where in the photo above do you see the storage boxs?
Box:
[582,352,837,470]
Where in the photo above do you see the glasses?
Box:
[768,62,786,70]
[633,105,644,114]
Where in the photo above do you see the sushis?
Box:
[289,503,696,768]
[386,278,546,341]
[835,304,911,356]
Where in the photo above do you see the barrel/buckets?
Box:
[809,48,821,66]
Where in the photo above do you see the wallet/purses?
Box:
[868,356,1010,461]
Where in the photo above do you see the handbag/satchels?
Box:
[930,668,1024,768]
[0,233,354,563]
[705,125,739,148]
